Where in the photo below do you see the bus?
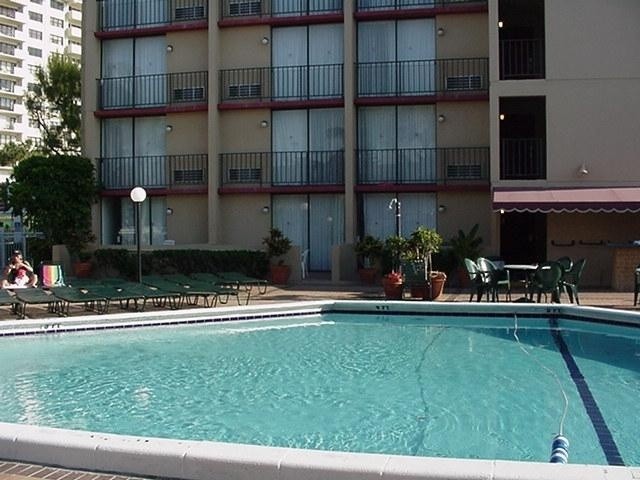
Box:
[0,203,38,232]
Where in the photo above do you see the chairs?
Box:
[299,248,311,281]
[459,250,589,309]
[0,270,272,320]
[633,268,640,307]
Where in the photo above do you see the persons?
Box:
[1,249,39,289]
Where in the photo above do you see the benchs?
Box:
[172,168,206,185]
[445,161,485,180]
[173,3,206,21]
[226,167,264,185]
[170,85,206,102]
[226,0,264,17]
[442,73,484,93]
[223,81,265,101]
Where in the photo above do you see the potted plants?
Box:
[260,226,295,285]
[349,220,487,300]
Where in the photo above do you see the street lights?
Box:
[130,186,149,285]
